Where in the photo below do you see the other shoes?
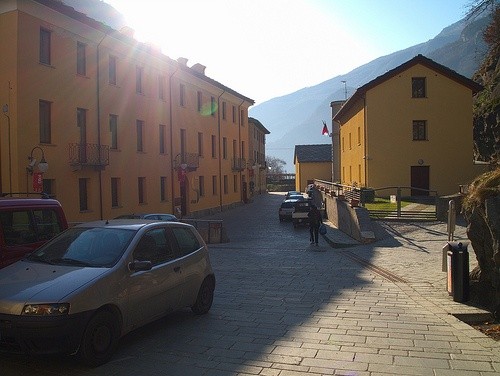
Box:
[310,241,318,246]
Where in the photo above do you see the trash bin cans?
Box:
[442,242,471,301]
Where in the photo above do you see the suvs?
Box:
[0,192,70,270]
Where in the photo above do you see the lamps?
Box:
[418,159,423,164]
[255,159,262,168]
[246,158,256,170]
[174,152,188,171]
[27,145,47,174]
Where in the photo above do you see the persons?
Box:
[307,205,322,246]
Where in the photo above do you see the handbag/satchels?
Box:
[320,223,327,235]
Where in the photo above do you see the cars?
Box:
[112,213,179,234]
[278,191,317,229]
[0,219,217,367]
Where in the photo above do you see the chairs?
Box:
[134,234,160,264]
[20,214,50,242]
[98,233,124,261]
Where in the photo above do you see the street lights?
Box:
[341,80,347,100]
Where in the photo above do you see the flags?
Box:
[321,122,329,135]
